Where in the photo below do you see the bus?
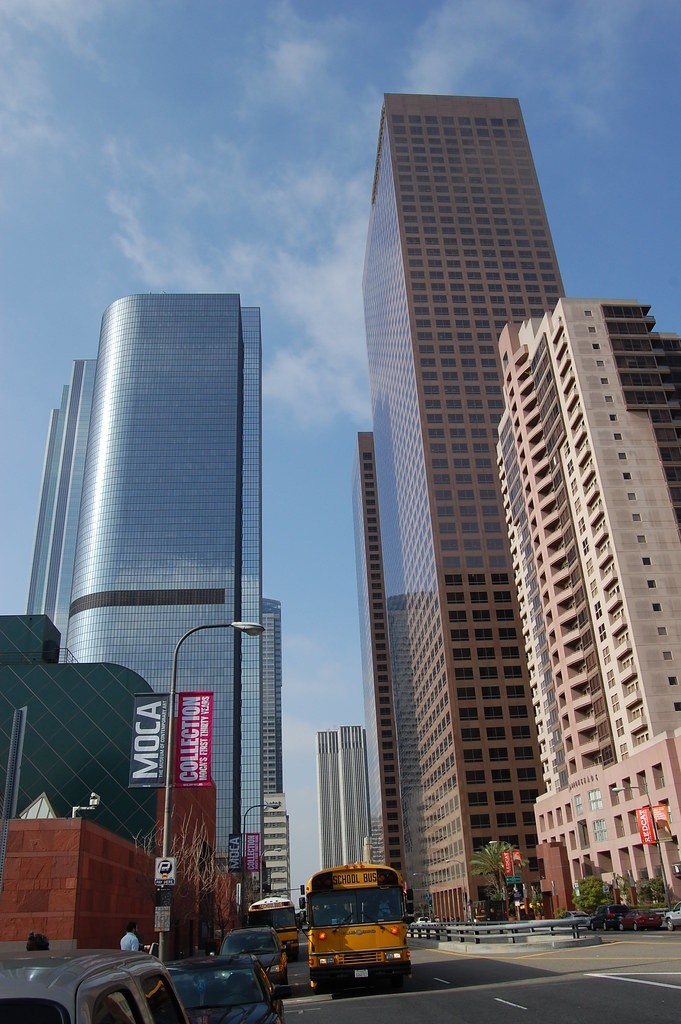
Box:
[299,862,415,994]
[245,896,303,956]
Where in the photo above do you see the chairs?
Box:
[378,902,391,916]
[224,973,262,1004]
[172,975,199,1007]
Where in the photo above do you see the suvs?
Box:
[209,925,291,985]
[162,953,293,1023]
[589,903,631,932]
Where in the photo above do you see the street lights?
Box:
[489,841,520,920]
[413,873,431,922]
[445,859,467,922]
[158,622,265,961]
[241,804,281,927]
[611,786,672,908]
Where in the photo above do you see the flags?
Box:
[634,803,674,844]
[127,692,171,789]
[172,691,216,788]
[228,833,244,872]
[246,832,261,872]
[502,851,522,876]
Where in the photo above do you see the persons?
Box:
[120,920,151,952]
[434,915,461,923]
[26,931,49,952]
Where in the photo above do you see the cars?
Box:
[618,909,663,931]
[556,911,590,920]
[415,917,431,924]
[664,901,681,932]
[0,948,190,1024]
